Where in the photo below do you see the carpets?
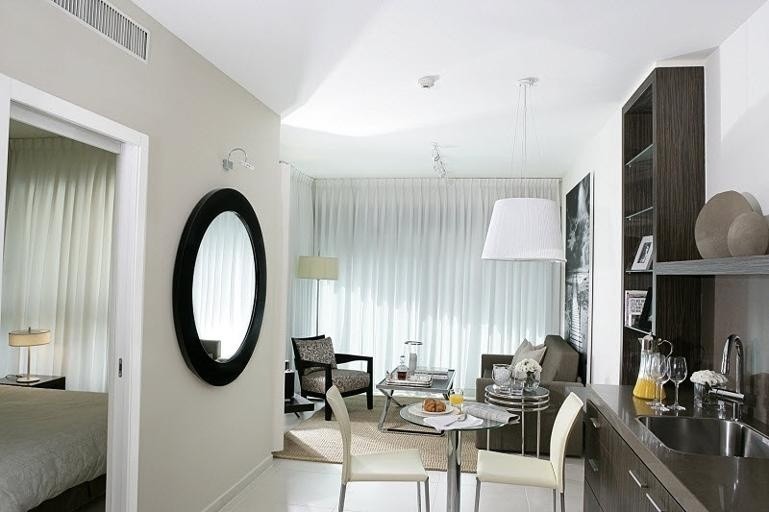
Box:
[271,394,478,472]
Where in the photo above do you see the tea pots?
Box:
[632,332,672,400]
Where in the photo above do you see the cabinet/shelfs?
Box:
[621,439,685,512]
[619,67,706,385]
[583,400,622,512]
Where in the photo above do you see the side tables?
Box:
[485,383,550,459]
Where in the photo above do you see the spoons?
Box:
[444,413,467,428]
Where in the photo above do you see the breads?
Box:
[424,398,446,411]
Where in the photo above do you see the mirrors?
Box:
[172,187,267,388]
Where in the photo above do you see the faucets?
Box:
[720,334,744,417]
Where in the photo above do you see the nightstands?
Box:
[0,374,65,391]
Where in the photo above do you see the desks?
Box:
[400,399,509,512]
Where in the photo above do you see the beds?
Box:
[0,384,108,512]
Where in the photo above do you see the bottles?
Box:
[397,355,407,381]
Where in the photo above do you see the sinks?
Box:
[635,415,769,458]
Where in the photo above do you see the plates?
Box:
[408,403,460,418]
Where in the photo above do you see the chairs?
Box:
[292,334,373,421]
[326,385,430,512]
[474,392,584,512]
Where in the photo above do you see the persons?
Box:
[639,243,650,262]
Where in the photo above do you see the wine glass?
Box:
[643,353,688,412]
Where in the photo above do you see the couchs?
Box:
[476,334,582,458]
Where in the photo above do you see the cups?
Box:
[492,364,540,395]
[450,390,463,415]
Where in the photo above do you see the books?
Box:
[624,289,648,328]
[413,363,450,381]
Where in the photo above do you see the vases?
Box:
[514,370,540,389]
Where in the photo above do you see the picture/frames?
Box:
[631,235,653,271]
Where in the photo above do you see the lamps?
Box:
[8,327,52,382]
[481,78,568,263]
[223,146,253,176]
[296,250,339,336]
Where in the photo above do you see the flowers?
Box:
[515,359,543,372]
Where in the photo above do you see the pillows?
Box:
[512,338,543,365]
[293,337,337,375]
[515,343,546,369]
